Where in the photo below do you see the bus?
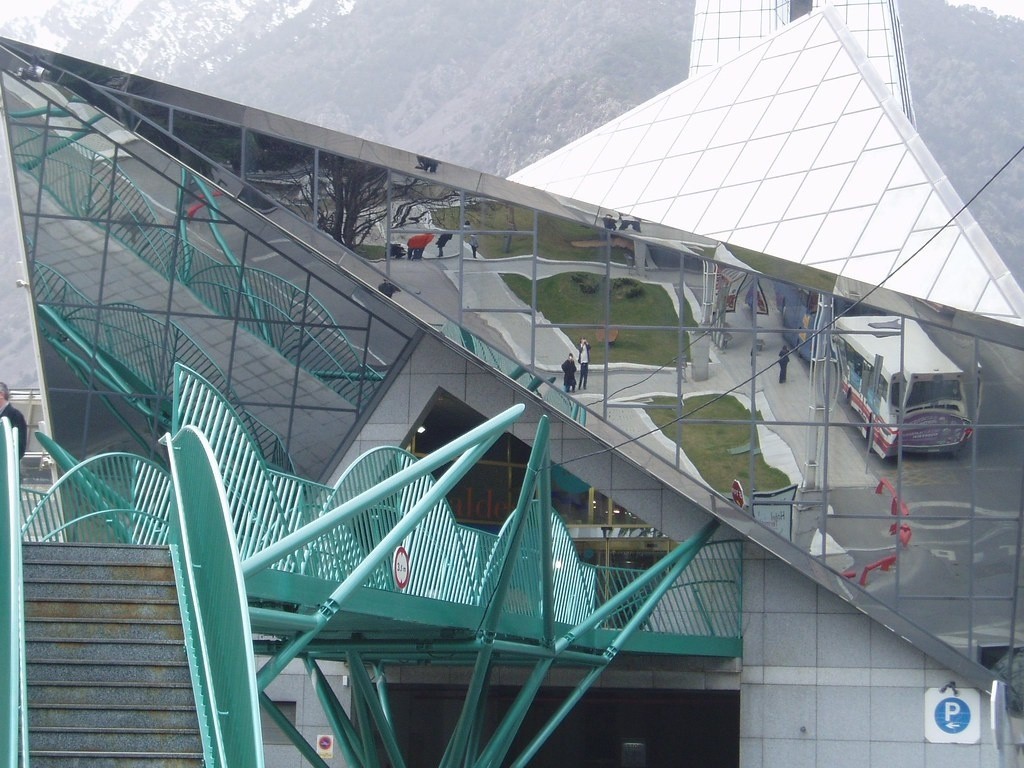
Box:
[773,281,887,362]
[834,316,972,459]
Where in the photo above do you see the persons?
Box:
[619,213,642,234]
[464,220,472,229]
[778,345,790,383]
[435,234,453,258]
[414,154,440,174]
[577,336,592,390]
[561,352,577,393]
[601,213,617,232]
[407,232,434,261]
[0,381,28,461]
[469,233,481,258]
